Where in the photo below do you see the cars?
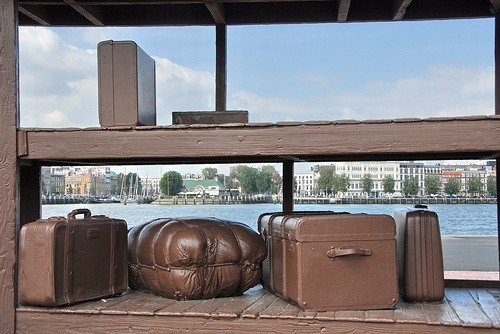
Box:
[293,192,497,199]
[42,193,161,200]
[242,194,283,200]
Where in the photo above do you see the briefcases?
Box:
[394,205,444,302]
[97,40,156,128]
[18,209,129,307]
